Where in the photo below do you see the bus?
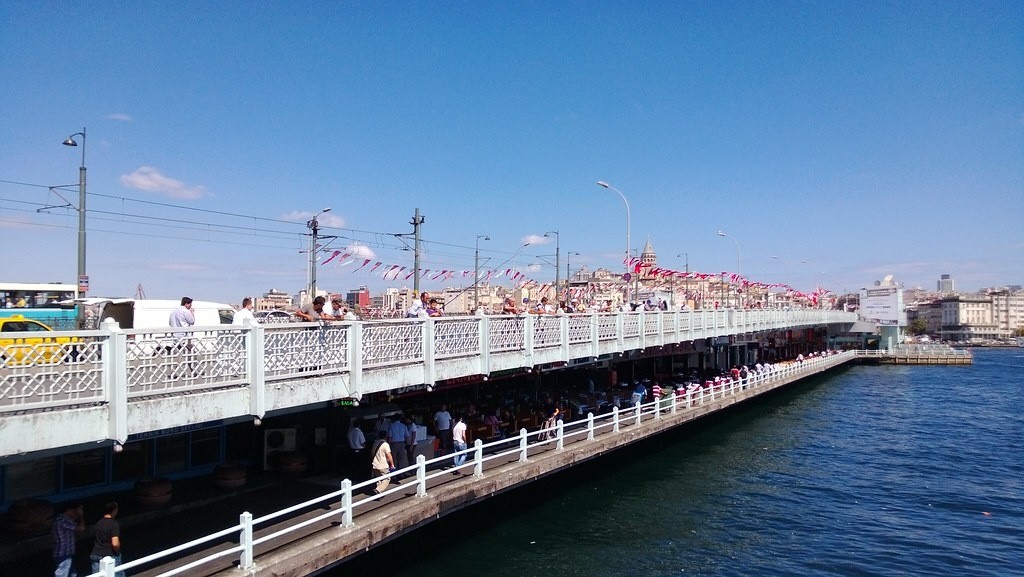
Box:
[0,282,77,331]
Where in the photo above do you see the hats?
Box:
[523,298,529,302]
[571,299,577,303]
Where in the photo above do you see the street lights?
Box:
[717,232,741,308]
[568,251,580,305]
[677,252,688,305]
[474,235,491,311]
[62,126,87,330]
[312,207,332,298]
[544,229,560,311]
[771,256,791,292]
[513,242,530,297]
[597,181,631,305]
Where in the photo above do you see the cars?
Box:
[900,333,1024,347]
[0,314,89,369]
[253,310,303,333]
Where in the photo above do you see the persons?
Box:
[370,412,419,495]
[636,381,647,405]
[652,382,667,400]
[50,502,85,577]
[406,292,442,317]
[294,296,356,371]
[634,299,667,312]
[467,404,514,447]
[6,296,26,309]
[90,501,126,577]
[796,348,839,362]
[231,299,258,376]
[601,300,613,313]
[169,297,204,378]
[348,422,367,482]
[501,297,585,348]
[433,403,467,476]
[543,397,559,438]
[715,362,771,389]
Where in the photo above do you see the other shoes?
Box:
[457,469,461,475]
[405,472,412,475]
[373,489,382,500]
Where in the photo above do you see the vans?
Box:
[50,297,238,360]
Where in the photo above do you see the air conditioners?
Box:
[264,429,297,471]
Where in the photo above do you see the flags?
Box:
[731,274,824,305]
[623,257,728,279]
